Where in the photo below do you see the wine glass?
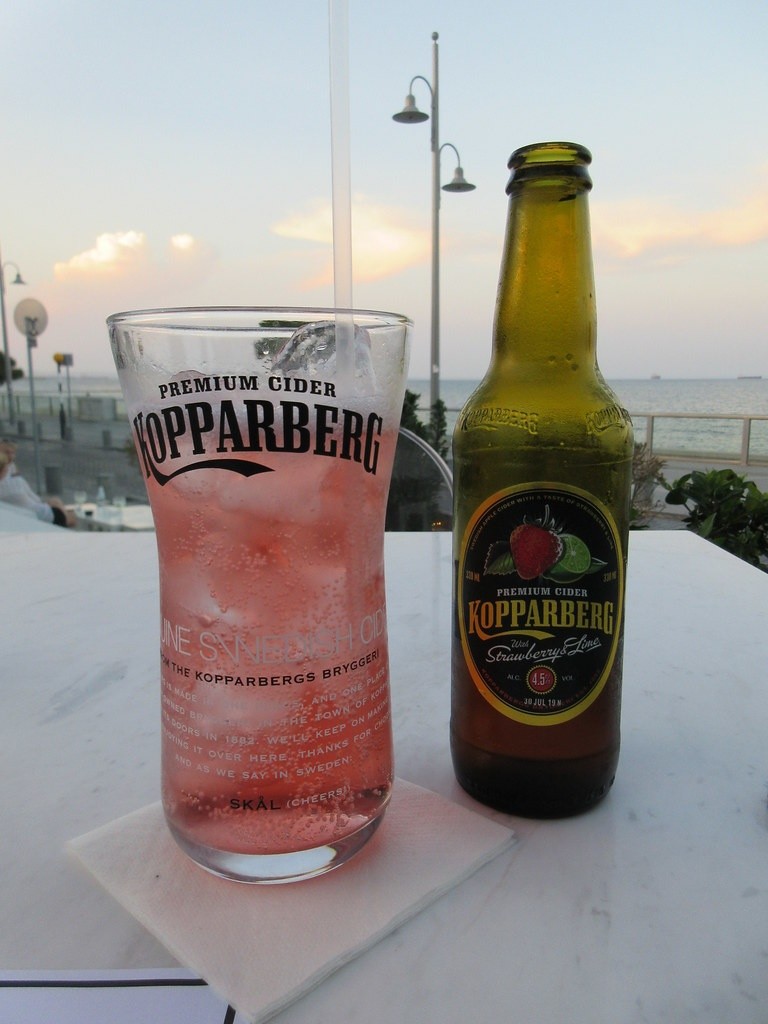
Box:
[74,491,87,513]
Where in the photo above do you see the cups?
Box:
[112,495,126,522]
[105,306,415,886]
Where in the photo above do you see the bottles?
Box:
[451,141,632,819]
[94,485,108,521]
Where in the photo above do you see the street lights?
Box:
[392,30,476,442]
[0,255,24,424]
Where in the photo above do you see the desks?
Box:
[1,529,767,1022]
